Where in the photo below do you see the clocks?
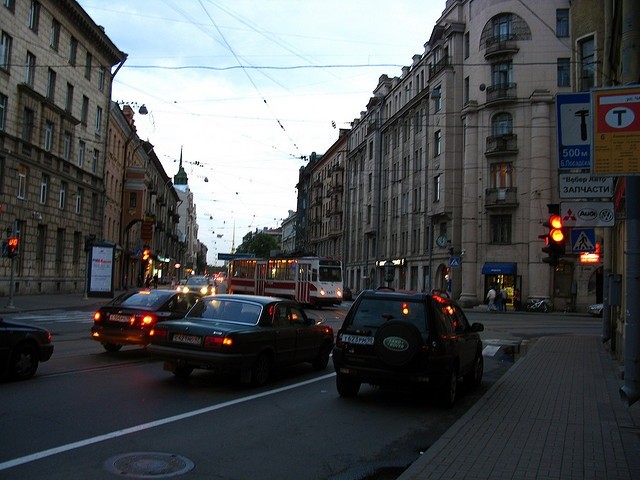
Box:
[436,235,447,249]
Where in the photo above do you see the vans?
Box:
[182,276,210,298]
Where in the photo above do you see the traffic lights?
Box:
[539,221,558,267]
[547,205,566,254]
[8,238,19,258]
[142,246,150,262]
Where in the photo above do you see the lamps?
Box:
[209,216,213,219]
[212,231,215,234]
[139,105,149,115]
[204,177,209,182]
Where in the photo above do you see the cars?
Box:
[1,319,55,382]
[90,288,204,353]
[208,281,219,294]
[174,279,187,293]
[148,293,335,387]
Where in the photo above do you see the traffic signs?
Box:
[554,91,591,170]
[558,172,614,198]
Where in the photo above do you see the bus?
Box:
[226,255,344,310]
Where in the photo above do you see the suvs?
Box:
[331,285,483,409]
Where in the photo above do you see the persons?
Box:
[486,286,496,312]
[502,287,508,309]
[145,273,150,288]
[513,288,520,308]
[494,289,503,312]
[153,273,158,290]
[170,274,176,290]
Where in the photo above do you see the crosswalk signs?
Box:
[571,228,596,252]
[449,256,461,268]
